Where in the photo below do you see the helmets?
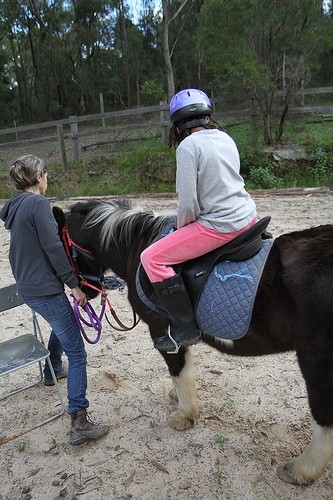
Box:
[169,89,213,135]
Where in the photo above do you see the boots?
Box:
[150,272,201,350]
[41,366,68,386]
[68,408,109,445]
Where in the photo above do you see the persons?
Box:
[0,153,112,448]
[137,89,260,352]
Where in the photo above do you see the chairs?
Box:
[0,282,65,446]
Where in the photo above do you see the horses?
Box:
[52,197,333,486]
